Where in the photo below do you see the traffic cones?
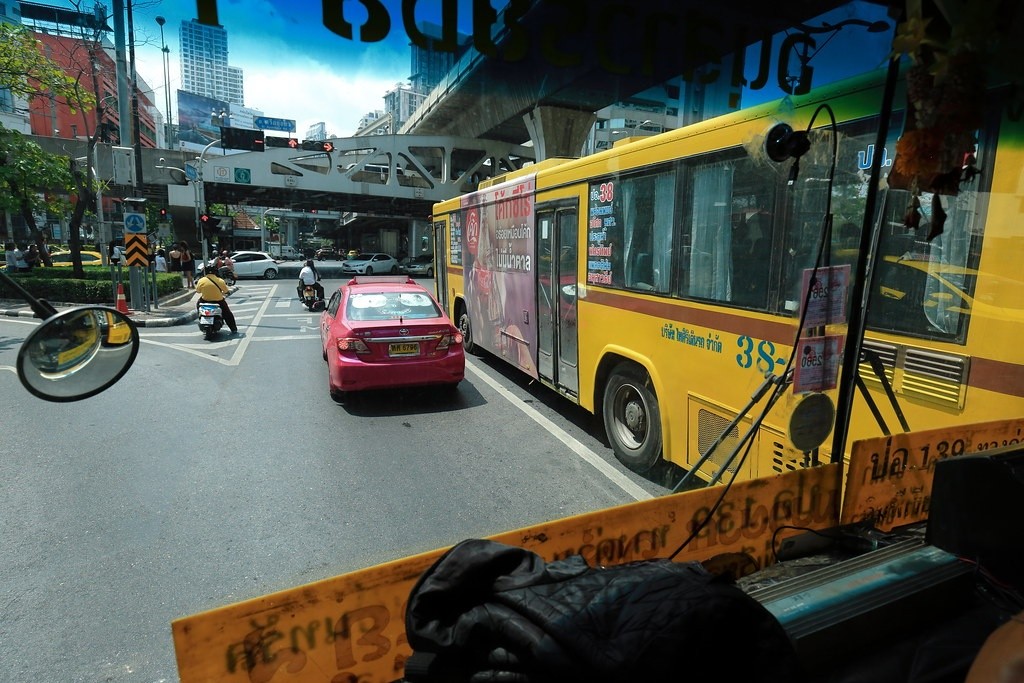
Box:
[115,283,134,316]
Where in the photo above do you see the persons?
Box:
[15,243,31,271]
[5,243,19,272]
[26,245,41,268]
[297,260,323,303]
[169,243,184,272]
[422,239,428,248]
[218,250,234,280]
[207,250,219,273]
[464,194,540,380]
[731,178,966,332]
[155,250,168,272]
[179,240,195,289]
[196,265,237,334]
[109,241,121,266]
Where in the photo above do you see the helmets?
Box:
[307,260,313,266]
[205,264,218,275]
[213,250,218,255]
[223,250,228,256]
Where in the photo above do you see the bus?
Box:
[432,61,1024,491]
[56,310,131,372]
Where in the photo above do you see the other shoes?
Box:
[232,327,237,333]
[300,297,305,304]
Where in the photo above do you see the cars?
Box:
[313,275,466,400]
[401,255,435,277]
[341,252,400,276]
[0,250,114,270]
[312,245,334,261]
[196,251,278,280]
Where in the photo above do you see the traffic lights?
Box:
[265,136,299,149]
[302,141,334,150]
[160,210,166,219]
[201,214,208,229]
[221,127,265,152]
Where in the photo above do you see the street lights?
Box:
[211,106,236,154]
[155,16,174,150]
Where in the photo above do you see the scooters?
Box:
[300,286,320,311]
[198,302,225,339]
[194,262,236,287]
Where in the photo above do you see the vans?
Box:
[268,245,304,261]
[347,163,404,175]
